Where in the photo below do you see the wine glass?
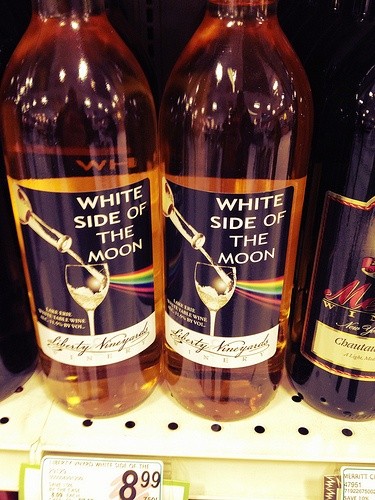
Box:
[65,263,110,336]
[194,261,237,336]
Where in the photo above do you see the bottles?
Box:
[13,183,72,254]
[163,178,206,251]
[158,1,314,426]
[286,0,375,424]
[0,0,165,419]
